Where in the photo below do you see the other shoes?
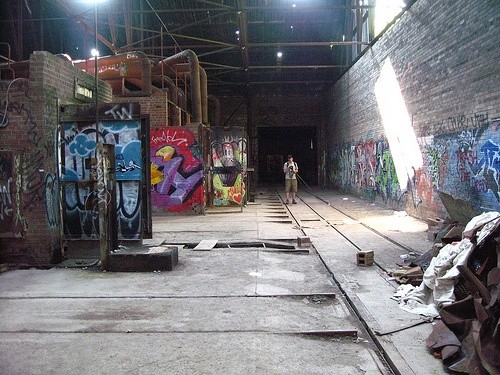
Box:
[286,201,290,204]
[292,201,297,204]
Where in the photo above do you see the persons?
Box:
[283,155,298,204]
